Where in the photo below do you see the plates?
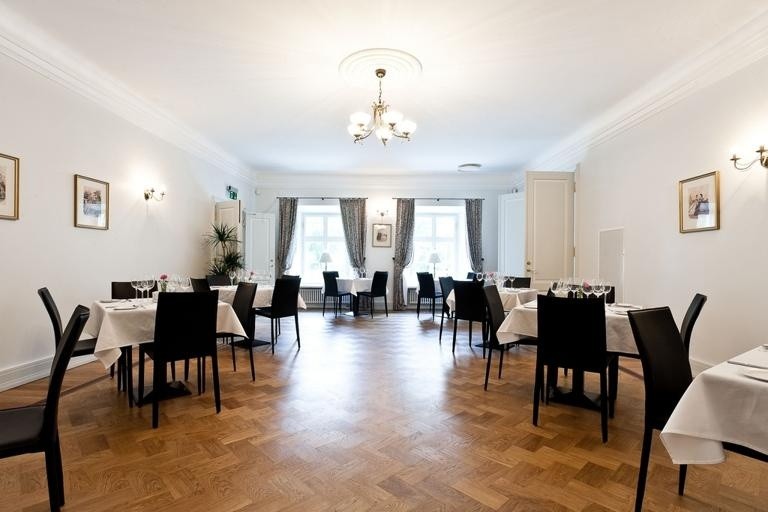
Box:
[746,368,768,382]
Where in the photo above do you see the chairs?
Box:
[0,304,91,511]
[38,287,127,394]
[184,285,299,350]
[111,280,176,393]
[189,276,212,292]
[182,281,258,382]
[250,275,301,355]
[205,273,232,286]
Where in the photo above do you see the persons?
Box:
[688,194,704,216]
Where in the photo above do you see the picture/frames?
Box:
[677,170,720,235]
[0,153,20,220]
[371,223,393,247]
[73,174,109,230]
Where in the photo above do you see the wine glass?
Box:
[99,275,191,312]
[345,267,369,279]
[228,269,251,286]
[476,272,643,315]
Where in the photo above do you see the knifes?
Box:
[729,359,768,370]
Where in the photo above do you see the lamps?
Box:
[144,188,166,202]
[319,253,332,270]
[429,253,441,276]
[339,47,424,151]
[730,145,768,170]
